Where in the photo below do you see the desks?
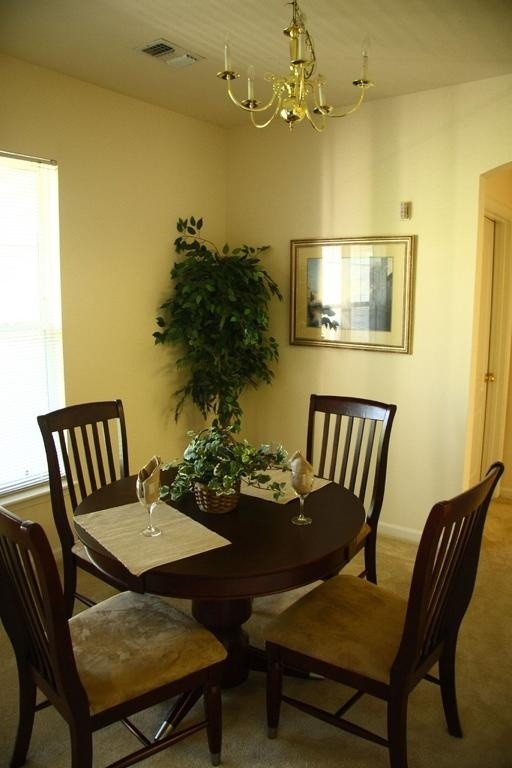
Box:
[75,465,368,741]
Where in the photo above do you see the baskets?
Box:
[193,429,241,513]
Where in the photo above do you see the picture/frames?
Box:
[288,234,415,352]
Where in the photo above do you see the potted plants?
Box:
[157,426,289,512]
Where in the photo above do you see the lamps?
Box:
[216,0,376,136]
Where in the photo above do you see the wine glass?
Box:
[135,478,162,538]
[289,469,314,527]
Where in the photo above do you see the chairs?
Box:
[37,398,144,619]
[272,394,397,614]
[1,503,229,768]
[260,459,505,767]
